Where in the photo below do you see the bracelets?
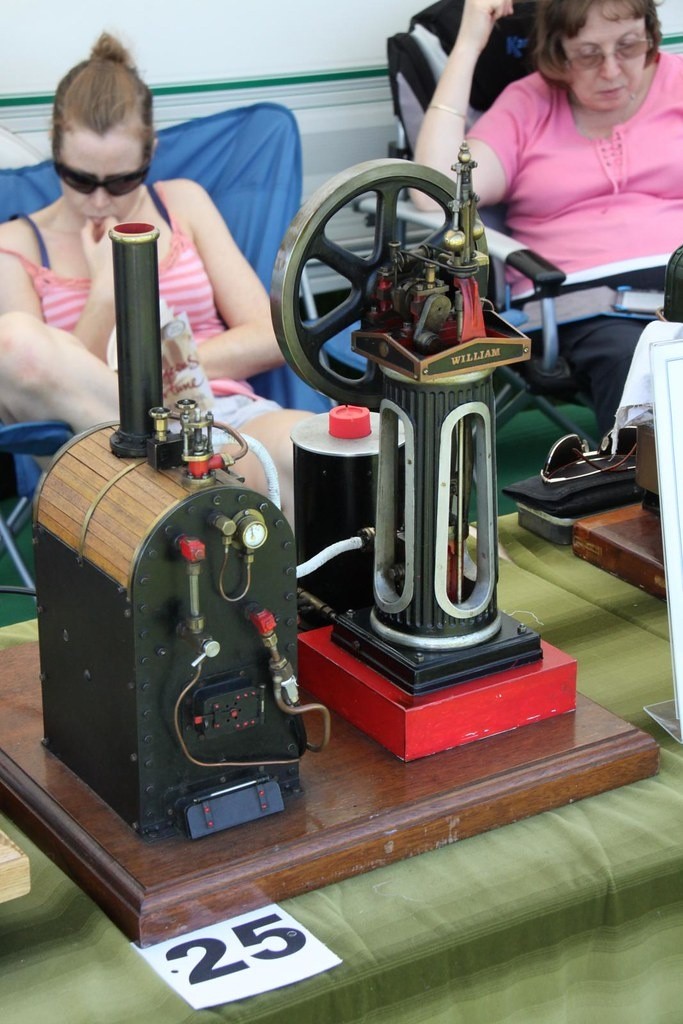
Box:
[428,104,467,120]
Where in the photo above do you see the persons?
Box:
[410,0,683,459]
[0,30,315,543]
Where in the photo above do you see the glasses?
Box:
[54,161,149,198]
[541,427,638,482]
[558,37,654,69]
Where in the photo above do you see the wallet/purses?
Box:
[503,453,646,519]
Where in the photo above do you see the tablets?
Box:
[615,286,666,314]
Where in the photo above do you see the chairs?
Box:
[352,0,590,445]
[0,100,338,600]
